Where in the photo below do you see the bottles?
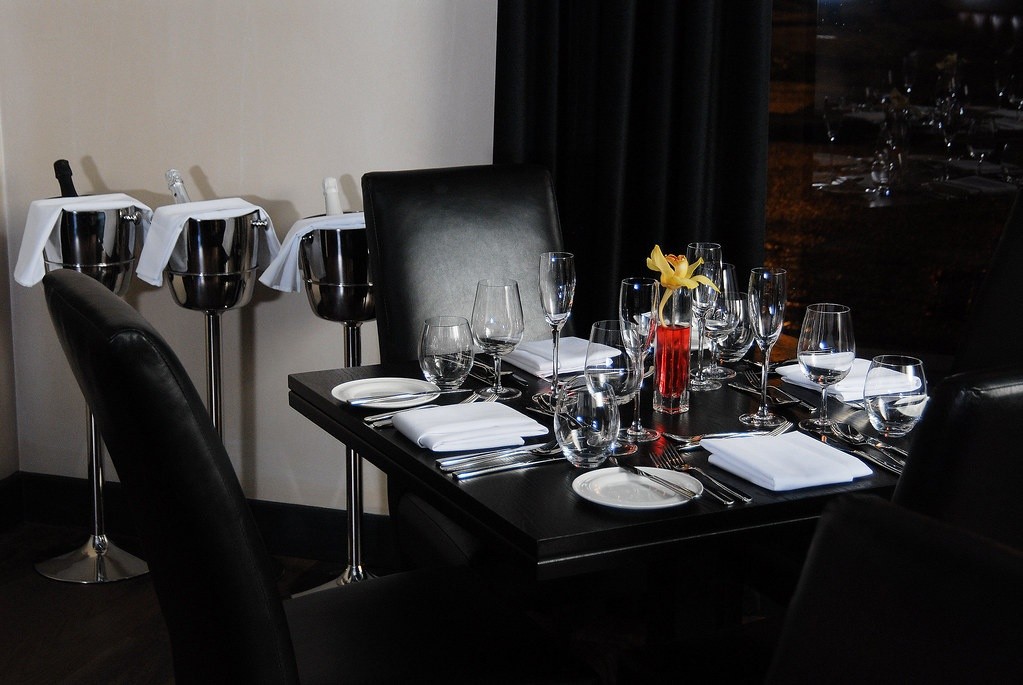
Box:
[53,159,78,197]
[322,177,343,216]
[165,167,190,204]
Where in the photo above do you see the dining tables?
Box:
[290,323,927,685]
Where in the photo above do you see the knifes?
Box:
[451,453,566,479]
[346,388,472,406]
[822,433,903,476]
[608,456,701,500]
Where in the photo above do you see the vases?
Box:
[651,284,693,415]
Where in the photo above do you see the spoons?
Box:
[835,422,906,467]
[727,381,801,406]
[439,438,558,470]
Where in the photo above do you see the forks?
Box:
[363,393,499,427]
[743,369,818,410]
[649,443,753,506]
[700,420,793,440]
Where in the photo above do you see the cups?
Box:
[704,292,754,363]
[554,382,621,466]
[863,355,927,437]
[417,315,475,390]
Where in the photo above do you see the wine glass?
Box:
[671,242,788,427]
[797,303,856,431]
[584,321,643,455]
[822,70,970,197]
[470,278,524,399]
[618,277,660,442]
[532,252,580,406]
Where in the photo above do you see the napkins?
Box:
[775,357,921,402]
[494,337,622,376]
[698,433,875,489]
[392,400,549,452]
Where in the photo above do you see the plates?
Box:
[572,466,703,509]
[331,377,441,408]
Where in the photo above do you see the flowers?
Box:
[644,244,721,329]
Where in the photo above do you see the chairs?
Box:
[42,163,1023,685]
